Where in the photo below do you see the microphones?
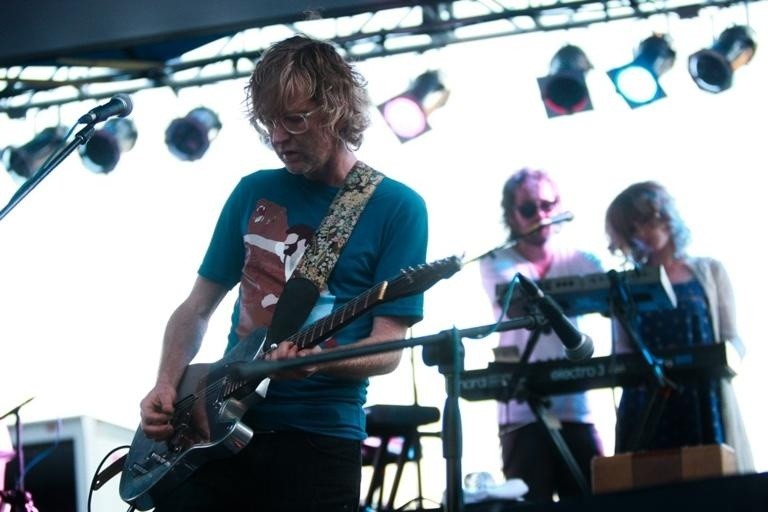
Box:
[539,208,576,231]
[516,271,595,366]
[80,89,138,125]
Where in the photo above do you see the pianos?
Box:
[461,340,739,400]
[494,264,677,321]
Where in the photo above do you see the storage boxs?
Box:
[592,444,735,494]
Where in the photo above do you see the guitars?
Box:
[120,255,462,511]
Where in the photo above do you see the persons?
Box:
[479,167,609,511]
[0,422,39,512]
[604,184,757,479]
[140,36,426,511]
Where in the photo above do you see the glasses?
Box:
[514,196,560,217]
[248,103,329,138]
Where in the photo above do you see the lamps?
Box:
[534,5,761,119]
[0,80,222,187]
[374,65,450,143]
